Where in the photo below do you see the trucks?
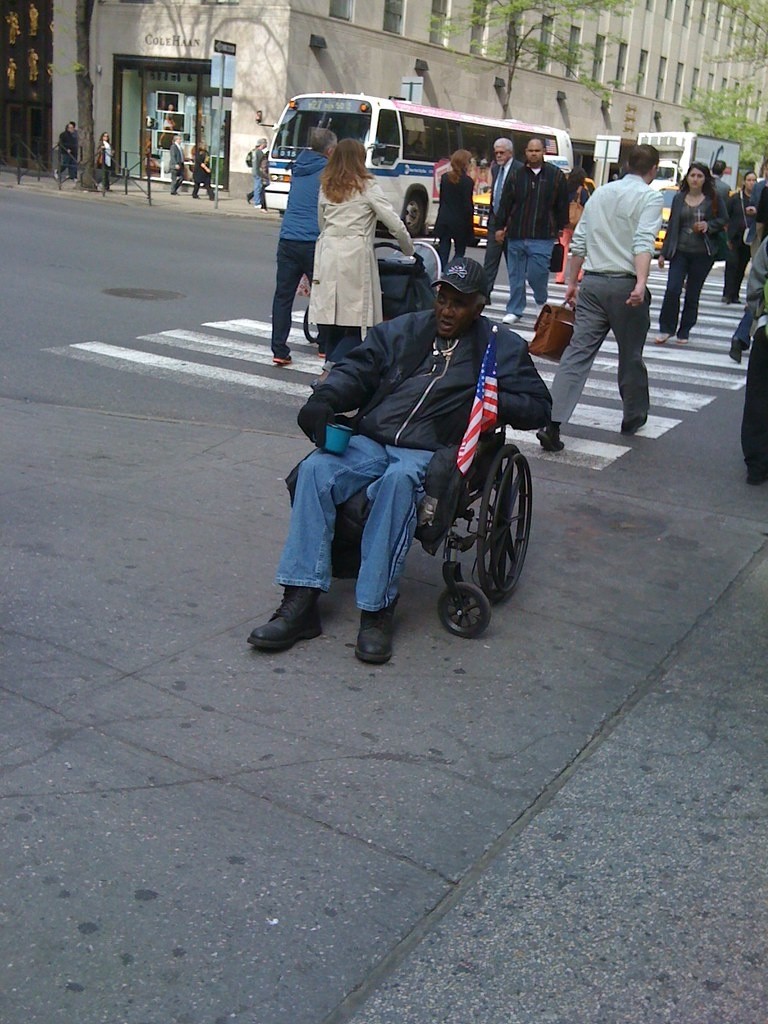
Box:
[635,132,741,192]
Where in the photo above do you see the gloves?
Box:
[296,394,336,448]
[435,400,471,445]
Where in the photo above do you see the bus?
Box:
[265,92,574,240]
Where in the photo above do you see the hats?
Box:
[430,257,492,297]
[256,138,267,146]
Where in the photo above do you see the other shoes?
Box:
[484,298,491,305]
[654,333,688,344]
[248,194,267,213]
[621,409,647,435]
[747,473,768,486]
[536,421,564,451]
[310,378,321,390]
[722,296,741,303]
[730,337,741,362]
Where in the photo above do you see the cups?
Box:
[694,213,704,233]
[312,423,353,454]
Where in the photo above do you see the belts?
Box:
[584,271,634,279]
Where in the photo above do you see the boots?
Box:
[355,592,400,663]
[247,586,322,651]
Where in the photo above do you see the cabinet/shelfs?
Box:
[146,90,198,184]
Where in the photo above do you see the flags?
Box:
[457,333,497,475]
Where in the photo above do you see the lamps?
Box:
[309,34,327,48]
[494,77,505,88]
[653,111,661,120]
[256,111,262,123]
[556,91,566,100]
[602,100,610,108]
[415,59,429,70]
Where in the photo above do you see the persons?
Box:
[495,137,570,324]
[308,138,416,389]
[169,135,185,196]
[270,128,338,364]
[192,142,215,200]
[711,158,768,483]
[655,162,730,344]
[95,132,116,192]
[248,139,270,213]
[158,104,175,146]
[555,166,593,284]
[434,149,475,276]
[483,138,525,305]
[536,144,664,450]
[53,121,78,182]
[247,254,553,663]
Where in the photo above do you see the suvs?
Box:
[471,173,597,248]
[655,185,737,251]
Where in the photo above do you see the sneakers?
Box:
[502,313,520,325]
[534,300,545,318]
[273,352,326,364]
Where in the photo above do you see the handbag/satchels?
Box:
[549,238,564,273]
[529,297,576,362]
[703,231,729,261]
[246,152,252,167]
[569,186,584,225]
[743,227,753,246]
[97,154,102,167]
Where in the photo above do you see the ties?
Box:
[493,166,504,215]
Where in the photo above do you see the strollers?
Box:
[302,242,443,343]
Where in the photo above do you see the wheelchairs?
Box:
[284,336,535,642]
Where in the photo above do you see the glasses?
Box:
[496,152,505,154]
